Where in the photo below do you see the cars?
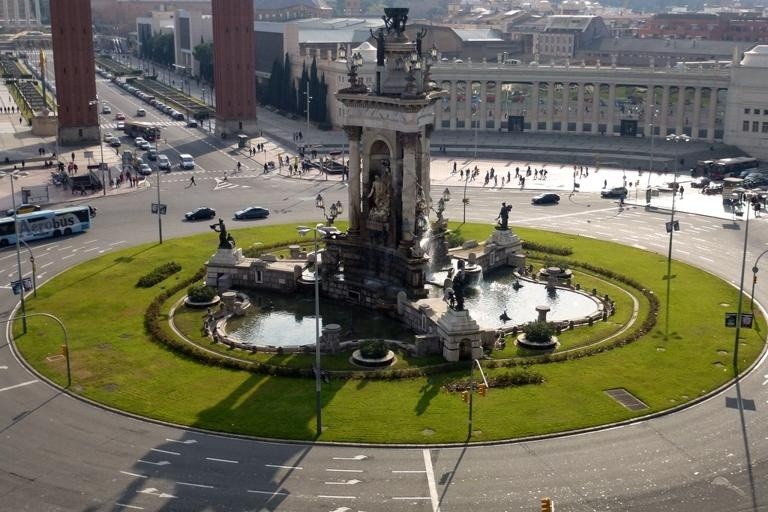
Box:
[104,132,171,175]
[185,207,216,220]
[5,204,40,216]
[691,170,768,205]
[601,187,628,197]
[117,121,125,129]
[187,120,197,128]
[531,193,560,203]
[234,206,270,217]
[97,68,184,120]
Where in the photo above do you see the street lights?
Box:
[296,223,341,434]
[727,187,768,365]
[665,134,691,257]
[429,188,450,223]
[474,99,482,157]
[316,194,342,227]
[0,170,29,333]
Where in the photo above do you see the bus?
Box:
[697,156,761,178]
[0,206,90,249]
[124,121,160,141]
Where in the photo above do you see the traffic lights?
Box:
[462,383,485,404]
[540,497,552,512]
[22,189,31,204]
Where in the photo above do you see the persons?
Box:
[453,276,464,310]
[370,28,384,66]
[440,145,746,201]
[227,233,235,245]
[416,27,427,57]
[448,292,456,306]
[213,218,231,249]
[755,201,760,217]
[0,105,348,196]
[498,203,511,227]
[367,175,387,211]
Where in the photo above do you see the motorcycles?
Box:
[90,208,96,217]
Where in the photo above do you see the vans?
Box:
[180,154,196,169]
